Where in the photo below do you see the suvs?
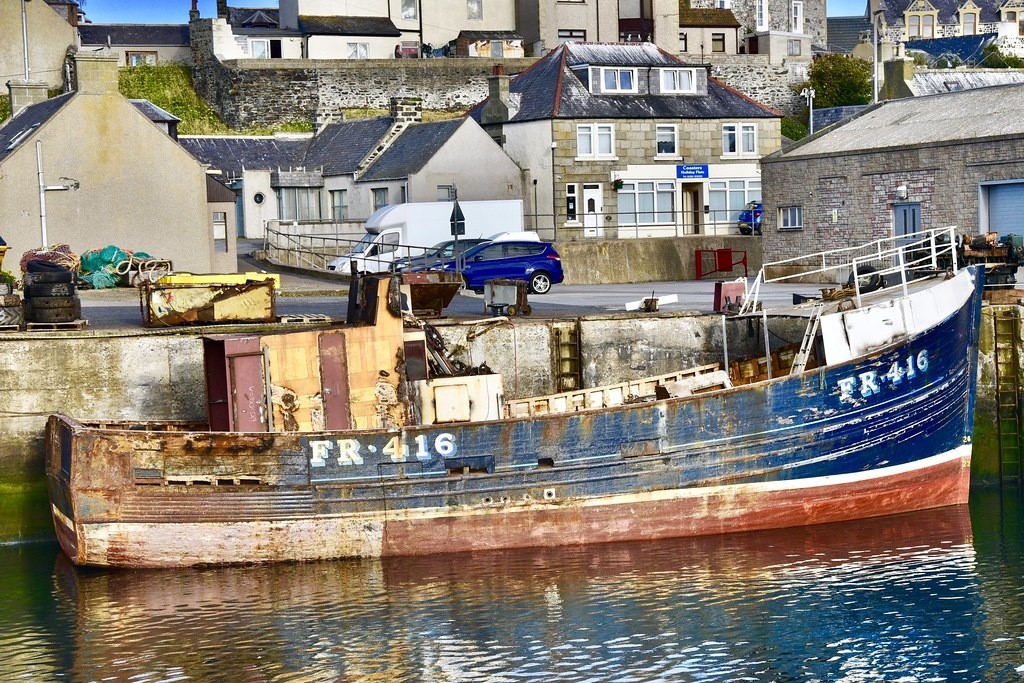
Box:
[410,239,564,294]
[387,238,493,274]
[738,200,761,235]
[490,230,543,242]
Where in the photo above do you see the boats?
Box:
[43,224,984,566]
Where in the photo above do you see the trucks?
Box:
[327,199,525,276]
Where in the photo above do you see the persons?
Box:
[426,43,434,58]
[395,45,402,58]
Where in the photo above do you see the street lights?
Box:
[872,4,886,105]
[799,86,814,136]
